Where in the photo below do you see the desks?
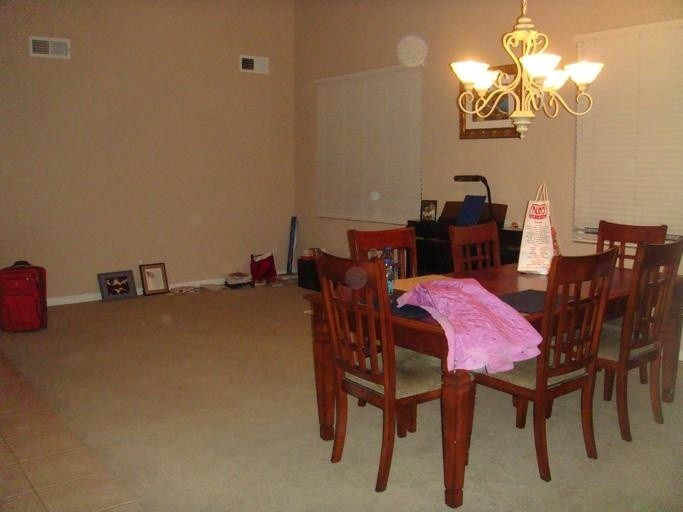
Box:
[305,265,683,508]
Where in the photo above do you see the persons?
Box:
[422,204,435,220]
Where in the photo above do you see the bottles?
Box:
[381,246,394,296]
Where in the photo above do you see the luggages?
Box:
[0,260,48,333]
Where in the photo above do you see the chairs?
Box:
[545,233,683,443]
[472,246,619,482]
[348,226,419,407]
[311,246,442,493]
[448,223,501,273]
[596,221,669,271]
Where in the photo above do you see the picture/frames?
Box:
[420,198,436,223]
[458,64,522,140]
[138,262,170,296]
[97,270,138,301]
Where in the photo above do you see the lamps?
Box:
[453,174,494,224]
[448,1,605,142]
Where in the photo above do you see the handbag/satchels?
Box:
[517,181,562,275]
